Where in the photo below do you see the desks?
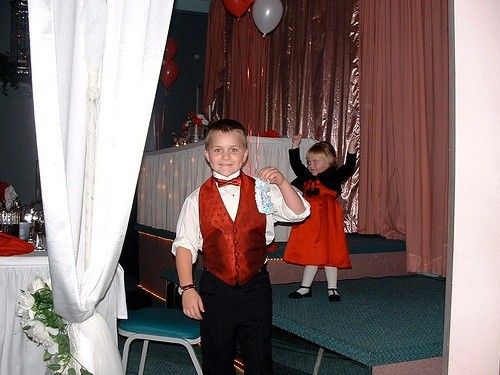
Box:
[0,251,128,375]
[136,136,321,242]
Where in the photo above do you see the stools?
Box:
[118,278,203,375]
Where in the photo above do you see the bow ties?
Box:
[214,176,241,188]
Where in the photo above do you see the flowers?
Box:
[14,275,93,375]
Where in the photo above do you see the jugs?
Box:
[0,211,20,238]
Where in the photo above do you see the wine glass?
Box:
[31,210,47,252]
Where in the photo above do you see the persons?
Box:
[171,119,311,375]
[288,133,358,302]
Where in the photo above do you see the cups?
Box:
[19,222,30,240]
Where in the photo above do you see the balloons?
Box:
[159,36,179,88]
[253,1,283,37]
[223,0,255,19]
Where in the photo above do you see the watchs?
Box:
[177,283,196,295]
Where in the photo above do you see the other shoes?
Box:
[288,286,312,298]
[328,288,340,302]
